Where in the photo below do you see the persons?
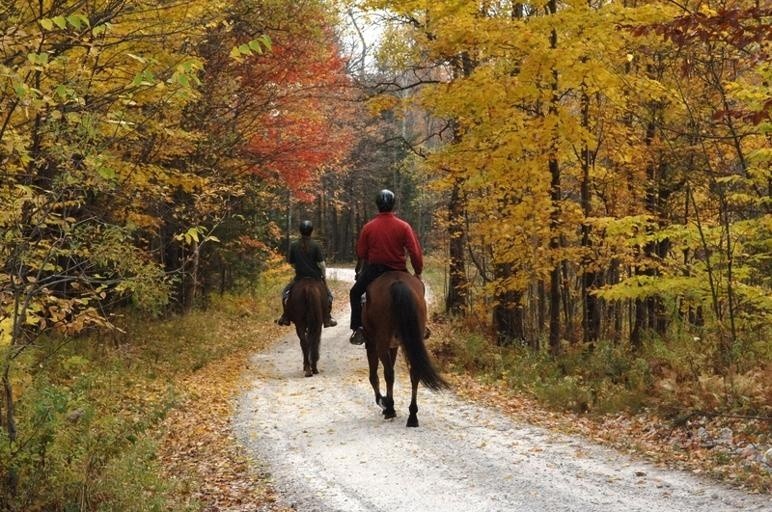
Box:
[348,188,425,346]
[276,219,337,327]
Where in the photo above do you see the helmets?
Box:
[299,220,314,234]
[375,189,396,212]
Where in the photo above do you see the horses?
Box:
[361,259,451,428]
[288,277,327,377]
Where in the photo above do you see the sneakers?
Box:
[281,313,291,327]
[324,318,337,328]
[350,326,365,343]
